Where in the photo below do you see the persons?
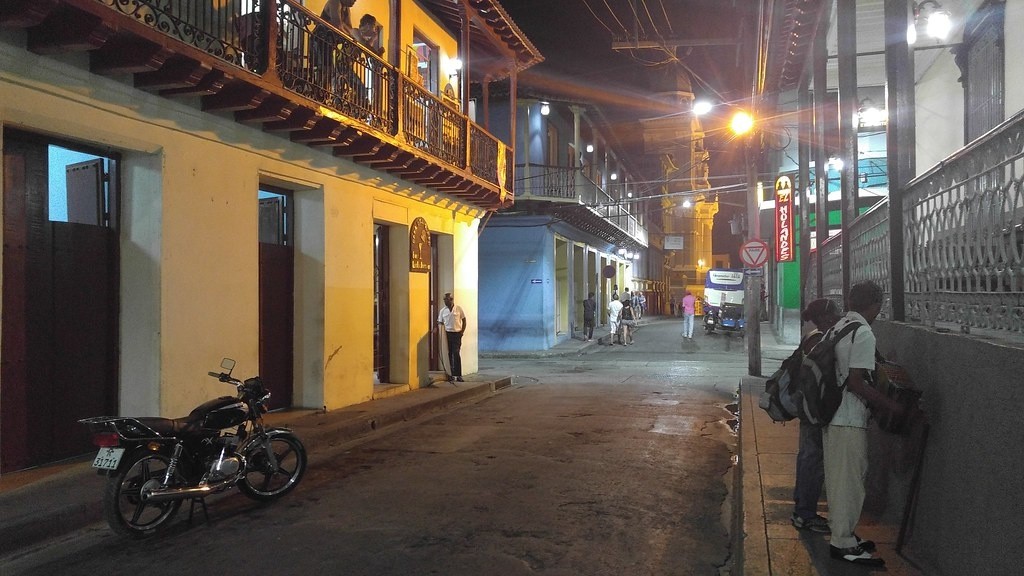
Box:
[606,295,636,346]
[790,299,842,533]
[819,281,907,567]
[682,291,695,339]
[611,285,646,319]
[583,292,596,342]
[437,293,466,382]
[310,0,385,119]
[760,286,767,321]
[237,11,284,71]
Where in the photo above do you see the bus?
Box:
[705,268,745,308]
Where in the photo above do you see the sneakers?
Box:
[829,532,886,567]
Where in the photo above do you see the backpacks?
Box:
[797,321,864,425]
[758,333,824,423]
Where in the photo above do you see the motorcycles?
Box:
[703,302,746,337]
[77,357,307,539]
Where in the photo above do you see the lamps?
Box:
[448,57,463,80]
[540,101,551,115]
[618,249,640,260]
[855,97,887,122]
[912,1,953,40]
[823,156,844,174]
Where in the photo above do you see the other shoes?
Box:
[456,377,465,382]
[587,338,594,341]
[583,336,588,341]
[624,343,627,346]
[791,508,832,535]
[630,342,634,345]
[681,332,688,337]
[688,335,693,339]
